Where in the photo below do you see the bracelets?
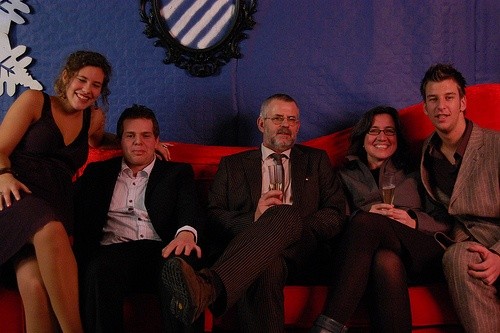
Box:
[0,166,13,175]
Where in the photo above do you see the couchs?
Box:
[1,83,500,331]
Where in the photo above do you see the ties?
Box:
[268,152,289,206]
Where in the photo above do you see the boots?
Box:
[161,256,218,326]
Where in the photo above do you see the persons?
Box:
[0,49,173,332]
[310,103,451,333]
[416,62,500,333]
[59,103,202,332]
[159,94,351,333]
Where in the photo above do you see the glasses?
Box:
[366,129,397,136]
[261,114,299,126]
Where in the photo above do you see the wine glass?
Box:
[268,164,283,206]
[381,175,396,205]
